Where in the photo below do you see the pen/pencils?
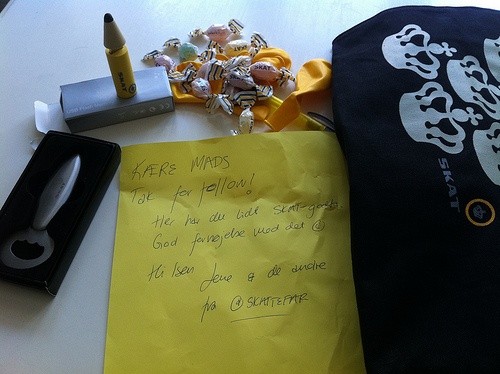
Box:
[264,91,336,138]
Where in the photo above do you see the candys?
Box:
[141,18,294,136]
